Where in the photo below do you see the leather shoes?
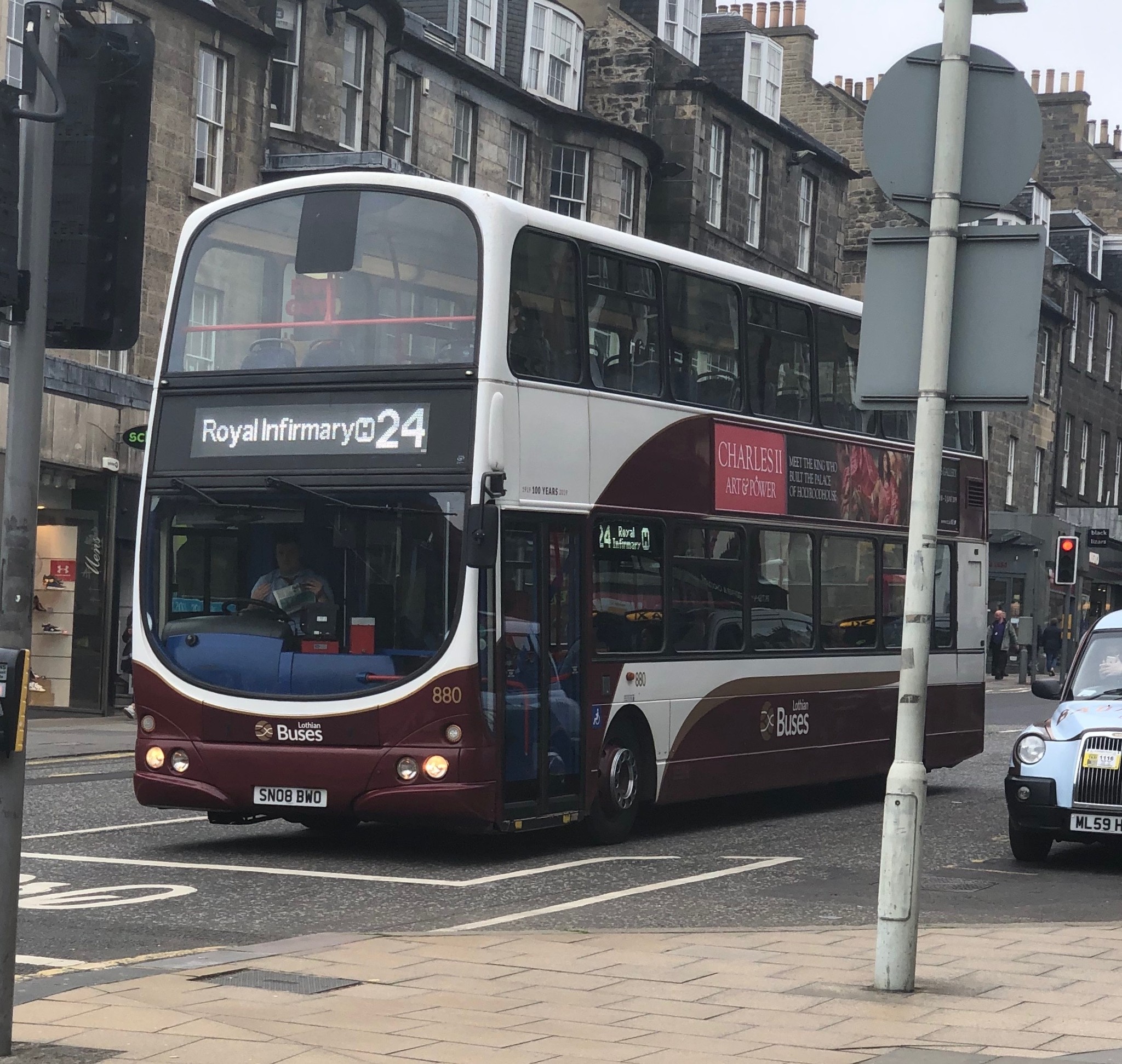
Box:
[33,596,46,611]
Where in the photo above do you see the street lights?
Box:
[869,0,1031,990]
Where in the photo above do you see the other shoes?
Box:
[996,677,1003,680]
[1048,672,1055,677]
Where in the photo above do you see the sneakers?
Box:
[43,573,66,590]
[123,704,135,718]
[28,681,46,691]
[40,622,62,635]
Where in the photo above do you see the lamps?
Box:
[324,0,369,23]
[785,150,818,167]
[657,162,688,180]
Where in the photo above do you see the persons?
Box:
[248,532,335,635]
[1099,654,1122,676]
[857,449,900,525]
[120,610,136,719]
[508,288,551,378]
[987,610,1061,679]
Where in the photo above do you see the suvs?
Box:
[681,606,835,651]
[835,612,951,648]
[1003,606,1122,864]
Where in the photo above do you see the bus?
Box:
[128,171,992,841]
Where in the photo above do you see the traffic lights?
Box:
[1053,535,1079,586]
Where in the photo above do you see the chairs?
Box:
[588,603,909,651]
[243,325,476,368]
[522,324,882,439]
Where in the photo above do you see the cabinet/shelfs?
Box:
[30,556,73,680]
